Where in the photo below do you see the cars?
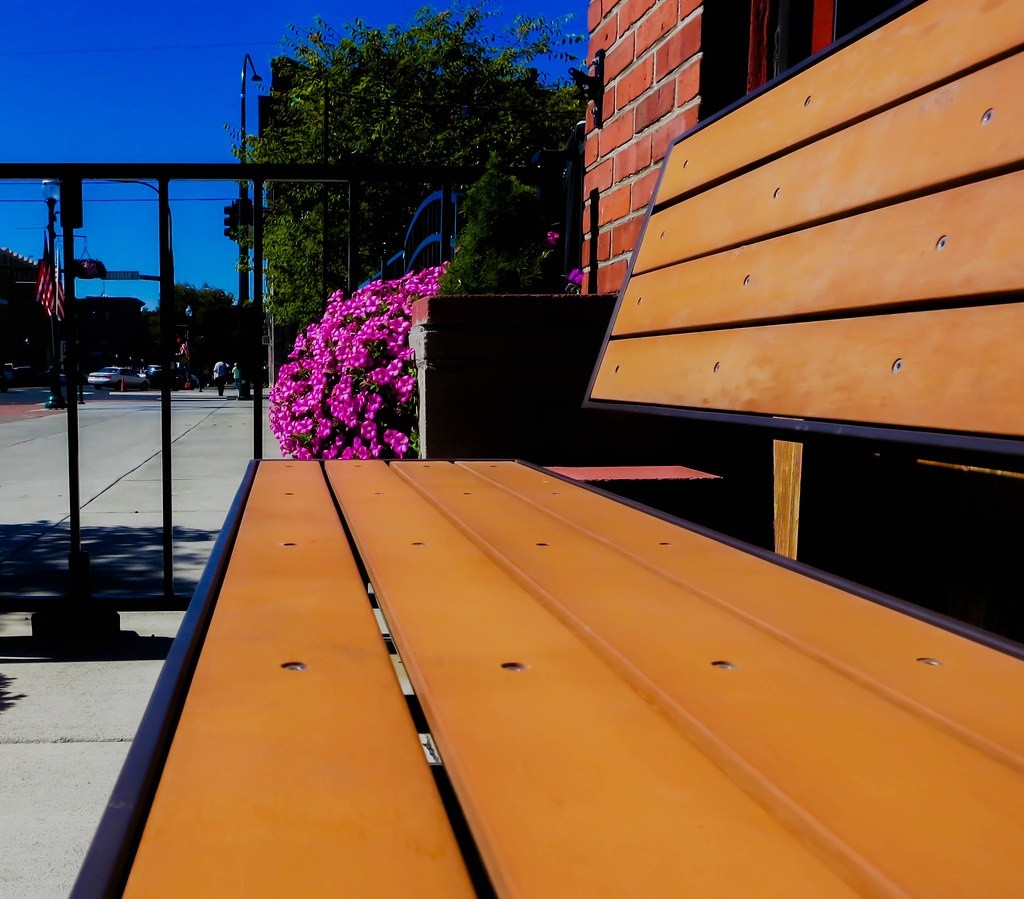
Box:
[0,359,67,388]
[86,361,149,390]
[145,365,200,392]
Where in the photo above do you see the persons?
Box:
[232,362,240,388]
[212,361,229,396]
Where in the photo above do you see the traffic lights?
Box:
[223,200,236,241]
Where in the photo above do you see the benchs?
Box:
[69,0,1024,899]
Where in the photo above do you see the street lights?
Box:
[40,180,64,407]
[185,302,194,392]
[237,54,264,401]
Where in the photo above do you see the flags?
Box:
[39,234,64,318]
